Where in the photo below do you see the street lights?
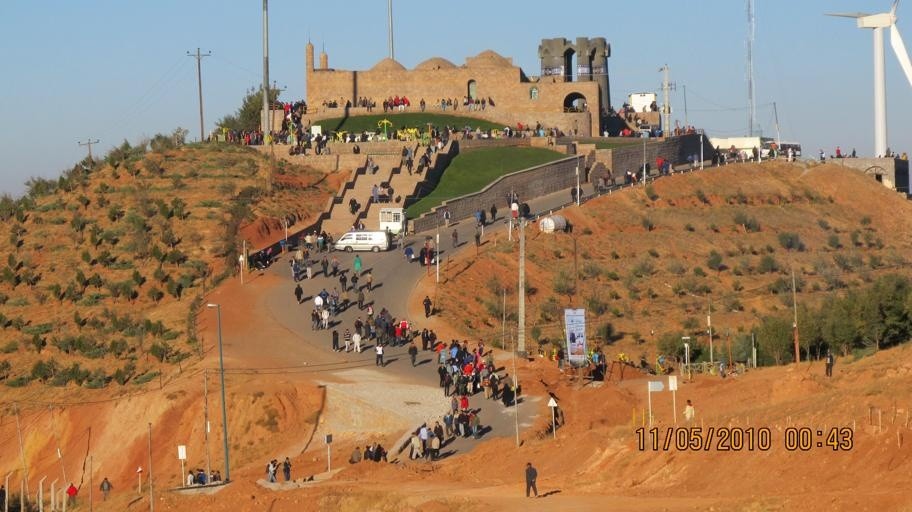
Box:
[681,336,690,367]
[206,299,230,486]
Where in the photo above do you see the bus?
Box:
[759,141,800,159]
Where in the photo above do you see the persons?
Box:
[526,463,538,497]
[408,329,517,463]
[349,182,437,267]
[66,482,77,509]
[825,351,834,379]
[423,296,432,318]
[752,142,906,162]
[292,229,333,253]
[0,485,5,508]
[251,239,287,271]
[100,478,113,501]
[290,249,409,367]
[571,101,747,202]
[559,348,565,370]
[350,442,388,464]
[206,94,561,176]
[443,190,520,247]
[188,468,223,486]
[267,457,290,482]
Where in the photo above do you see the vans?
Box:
[333,229,391,254]
